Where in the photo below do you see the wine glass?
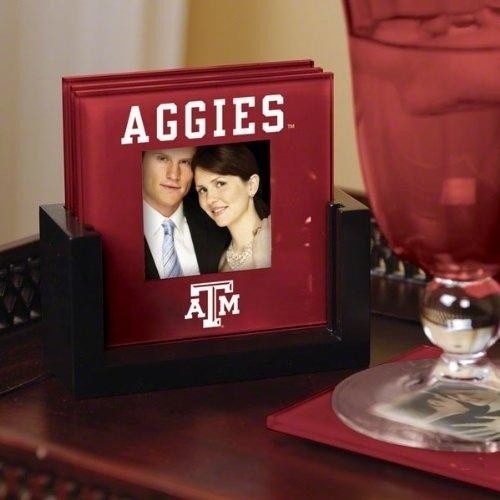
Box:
[330,0,499,454]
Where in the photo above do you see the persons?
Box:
[192,144,273,272]
[142,144,218,281]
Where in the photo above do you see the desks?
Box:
[0,303,500,499]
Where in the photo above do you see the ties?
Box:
[162,219,183,277]
